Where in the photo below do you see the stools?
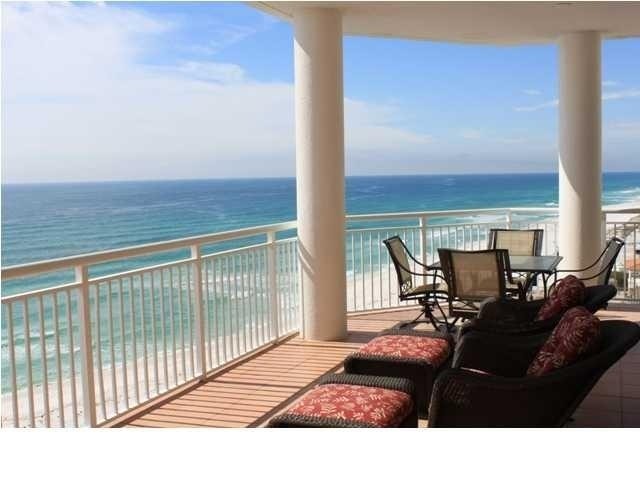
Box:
[267,372,418,428]
[343,328,451,420]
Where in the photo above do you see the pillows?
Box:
[526,307,606,381]
[533,273,587,321]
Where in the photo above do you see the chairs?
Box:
[436,244,526,331]
[383,235,454,333]
[544,238,626,325]
[427,319,640,426]
[457,284,617,344]
[489,227,544,302]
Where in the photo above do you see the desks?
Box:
[427,255,563,323]
